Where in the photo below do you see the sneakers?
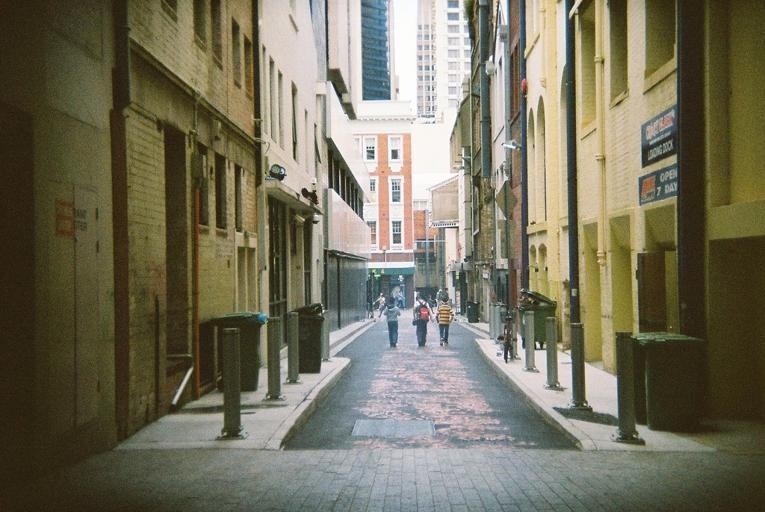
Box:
[389,337,448,348]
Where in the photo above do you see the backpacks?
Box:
[417,299,430,323]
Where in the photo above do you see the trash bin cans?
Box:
[291,302,325,375]
[201,313,261,392]
[467,301,480,323]
[630,330,705,432]
[521,292,557,344]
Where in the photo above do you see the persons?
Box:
[436,288,442,310]
[435,296,454,346]
[427,294,435,314]
[373,292,386,318]
[397,288,406,309]
[383,298,401,348]
[412,292,435,346]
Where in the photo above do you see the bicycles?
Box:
[493,302,524,364]
